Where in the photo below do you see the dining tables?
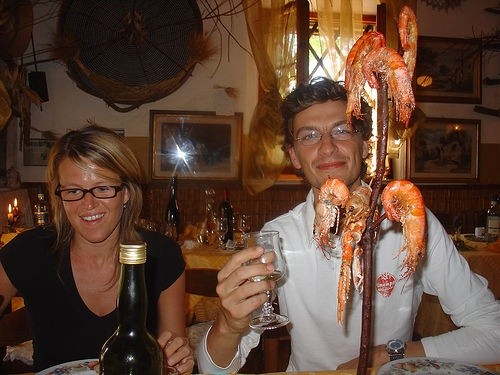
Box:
[415,232,500,338]
[181,233,249,325]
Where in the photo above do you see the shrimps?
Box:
[309,178,350,261]
[336,185,371,327]
[343,30,416,130]
[381,179,426,294]
[397,5,418,78]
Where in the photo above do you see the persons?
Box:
[194,76,500,375]
[0,121,196,375]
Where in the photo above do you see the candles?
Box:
[12,198,19,217]
[7,204,14,225]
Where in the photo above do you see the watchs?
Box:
[385,339,407,361]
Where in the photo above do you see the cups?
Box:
[474,227,485,238]
[136,218,178,240]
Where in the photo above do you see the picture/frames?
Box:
[404,116,482,185]
[474,37,500,116]
[411,35,483,105]
[147,108,244,182]
[24,138,53,168]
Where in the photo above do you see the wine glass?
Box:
[241,230,290,330]
[238,214,252,247]
[214,217,228,249]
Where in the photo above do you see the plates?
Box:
[35,358,100,375]
[377,356,500,375]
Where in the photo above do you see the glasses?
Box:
[55,183,126,201]
[290,125,362,145]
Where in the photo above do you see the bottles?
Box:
[32,193,50,228]
[486,201,500,240]
[98,241,164,375]
[164,175,181,241]
[196,188,221,247]
[218,190,234,248]
